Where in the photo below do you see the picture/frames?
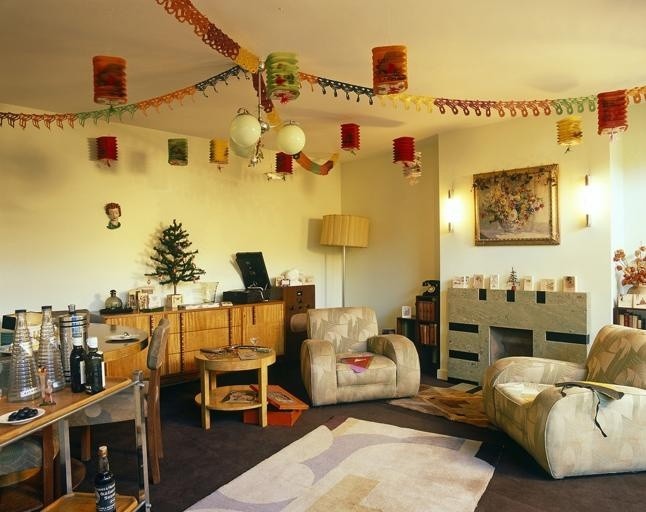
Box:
[250,384,308,410]
[473,163,559,247]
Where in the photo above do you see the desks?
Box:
[1,316,152,512]
[194,344,279,429]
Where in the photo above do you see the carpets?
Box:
[183,417,494,512]
[388,383,493,427]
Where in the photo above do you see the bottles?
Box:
[94,445,117,511]
[68,336,106,394]
[6,301,66,399]
[103,289,122,308]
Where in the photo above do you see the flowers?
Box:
[614,246,646,285]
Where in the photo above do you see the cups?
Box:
[199,281,218,303]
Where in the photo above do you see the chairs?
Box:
[38,316,169,499]
[301,308,421,405]
[486,324,646,481]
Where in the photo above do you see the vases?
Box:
[627,285,646,305]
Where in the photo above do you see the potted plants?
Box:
[144,219,205,307]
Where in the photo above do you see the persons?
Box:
[103,201,122,230]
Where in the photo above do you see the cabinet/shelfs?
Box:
[100,302,286,385]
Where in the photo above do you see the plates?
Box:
[0,407,46,426]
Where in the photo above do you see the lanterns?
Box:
[96,135,119,168]
[341,123,361,158]
[91,53,128,107]
[403,150,424,186]
[372,45,409,96]
[556,113,583,153]
[596,89,628,141]
[392,136,415,169]
[275,151,294,183]
[168,136,188,167]
[209,136,231,173]
[264,49,301,106]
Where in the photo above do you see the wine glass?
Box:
[249,337,260,355]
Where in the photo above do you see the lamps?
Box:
[321,214,370,306]
[226,62,305,165]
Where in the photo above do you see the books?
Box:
[397,300,440,346]
[198,342,271,407]
[127,285,163,311]
[614,293,646,330]
